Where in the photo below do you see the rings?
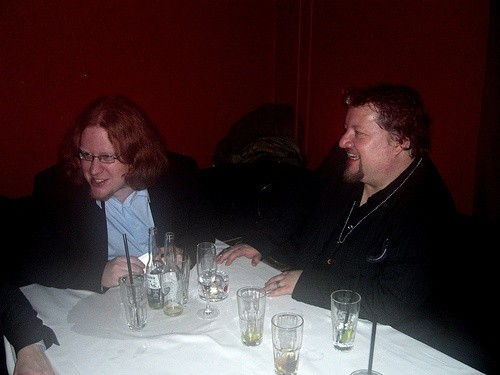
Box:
[122,277,126,284]
[219,254,223,256]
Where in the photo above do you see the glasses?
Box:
[79,151,124,163]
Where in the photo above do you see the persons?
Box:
[26,93,213,292]
[214,84,496,372]
[0,281,56,375]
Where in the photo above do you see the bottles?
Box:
[146,227,182,317]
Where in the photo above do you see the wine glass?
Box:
[197,242,219,320]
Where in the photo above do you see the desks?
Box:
[4,247,487,375]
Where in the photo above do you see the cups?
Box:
[173,253,189,304]
[198,271,230,302]
[330,289,361,352]
[271,312,304,375]
[237,286,266,347]
[118,274,148,331]
[350,368,382,375]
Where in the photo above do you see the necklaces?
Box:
[325,156,423,265]
